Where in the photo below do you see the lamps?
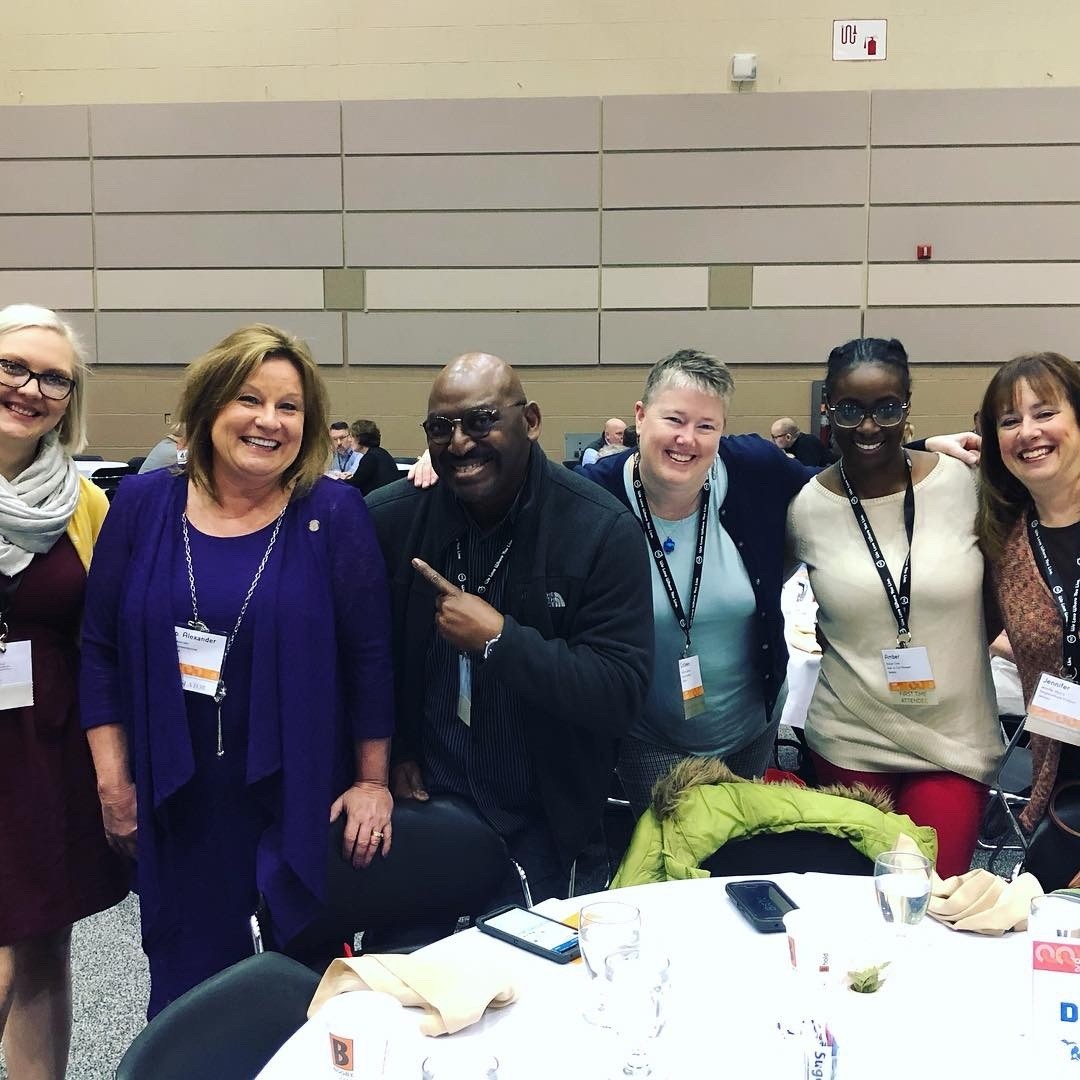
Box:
[730,52,758,81]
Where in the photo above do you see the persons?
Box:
[786,337,1005,880]
[363,351,655,913]
[598,426,640,462]
[138,422,189,475]
[579,417,628,469]
[329,422,365,480]
[967,352,1080,900]
[409,349,984,806]
[0,303,110,1080]
[81,323,396,1026]
[334,420,400,497]
[769,417,832,470]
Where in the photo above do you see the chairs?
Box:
[115,950,324,1080]
[257,801,532,976]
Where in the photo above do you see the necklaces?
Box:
[184,505,288,759]
[645,494,699,554]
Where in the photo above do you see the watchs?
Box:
[484,634,501,659]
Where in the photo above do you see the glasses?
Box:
[420,402,527,445]
[333,433,351,442]
[772,433,789,444]
[0,359,76,401]
[824,394,909,429]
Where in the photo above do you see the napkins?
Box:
[895,834,1048,938]
[305,953,521,1039]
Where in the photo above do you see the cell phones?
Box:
[725,880,800,932]
[477,904,581,964]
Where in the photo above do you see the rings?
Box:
[372,832,383,837]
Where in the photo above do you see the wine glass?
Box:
[605,949,670,1080]
[578,902,642,1028]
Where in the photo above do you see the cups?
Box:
[421,1050,500,1080]
[782,909,844,991]
[1031,893,1080,929]
[322,991,402,1080]
[874,852,933,945]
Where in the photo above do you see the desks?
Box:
[256,870,1080,1080]
